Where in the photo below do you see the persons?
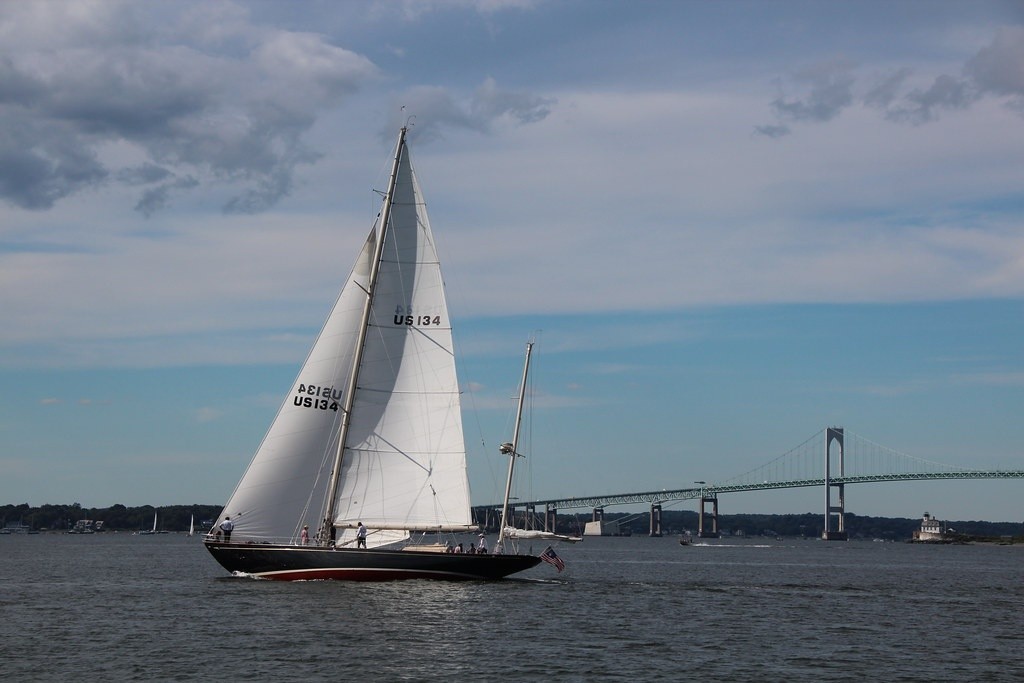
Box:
[301,525,310,546]
[356,521,367,549]
[468,543,476,553]
[219,516,234,543]
[454,543,463,553]
[446,545,454,553]
[329,522,336,548]
[477,534,488,554]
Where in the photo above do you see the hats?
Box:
[478,534,484,537]
[304,525,309,527]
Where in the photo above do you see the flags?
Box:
[539,545,565,573]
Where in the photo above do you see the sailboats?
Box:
[204,107,566,577]
[187,514,198,536]
[139,511,169,534]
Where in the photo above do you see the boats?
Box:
[505,525,584,544]
[681,531,692,544]
[68,523,94,533]
